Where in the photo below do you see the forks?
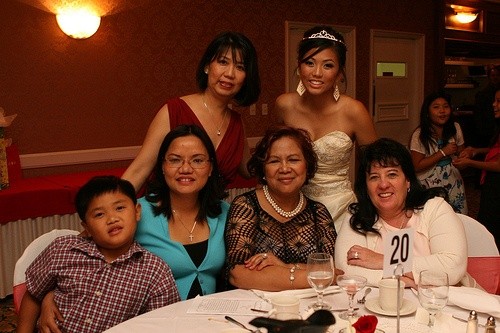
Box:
[357,288,372,304]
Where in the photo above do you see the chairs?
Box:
[11,229,81,328]
[453,256,500,296]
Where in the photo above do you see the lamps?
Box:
[451,5,478,23]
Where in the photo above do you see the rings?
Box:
[262,254,266,258]
[355,254,357,258]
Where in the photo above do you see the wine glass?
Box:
[417,270,449,329]
[336,274,368,321]
[306,253,334,311]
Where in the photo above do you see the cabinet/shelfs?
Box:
[445,60,476,117]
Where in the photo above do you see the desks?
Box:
[0,166,267,300]
[102,286,500,333]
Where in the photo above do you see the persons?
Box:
[38,125,231,333]
[453,83,500,235]
[16,175,181,333]
[411,92,469,215]
[223,128,337,292]
[273,26,376,233]
[120,31,262,195]
[335,138,468,289]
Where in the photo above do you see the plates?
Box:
[365,295,417,316]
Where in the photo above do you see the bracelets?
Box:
[440,149,446,156]
[290,267,295,289]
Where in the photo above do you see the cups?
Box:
[378,279,405,312]
[267,296,300,321]
[339,326,385,333]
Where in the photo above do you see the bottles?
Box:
[466,310,478,333]
[485,317,496,333]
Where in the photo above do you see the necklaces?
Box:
[201,93,222,135]
[173,210,197,241]
[263,185,303,218]
